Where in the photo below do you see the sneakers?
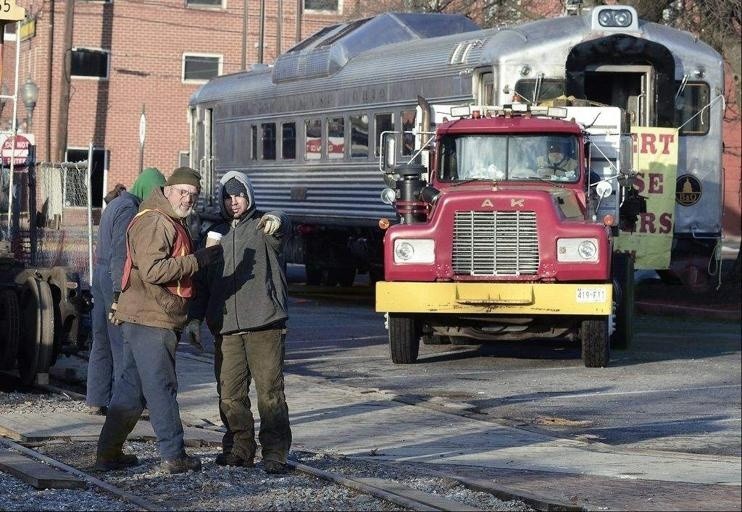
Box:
[161,455,189,474]
[215,451,257,468]
[95,450,138,472]
[88,404,108,416]
[262,459,286,473]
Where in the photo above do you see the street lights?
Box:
[19,72,42,265]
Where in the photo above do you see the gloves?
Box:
[255,214,283,236]
[194,242,225,271]
[185,319,206,353]
[107,291,127,327]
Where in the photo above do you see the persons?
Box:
[185,171,292,475]
[525,144,600,186]
[87,168,167,420]
[95,166,224,474]
[104,184,126,204]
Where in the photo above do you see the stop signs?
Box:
[1,133,35,174]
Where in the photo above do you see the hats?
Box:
[164,166,201,194]
[221,178,249,202]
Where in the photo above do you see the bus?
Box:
[186,2,728,300]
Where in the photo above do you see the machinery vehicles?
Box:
[372,73,635,369]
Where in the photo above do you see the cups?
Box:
[204,231,222,249]
